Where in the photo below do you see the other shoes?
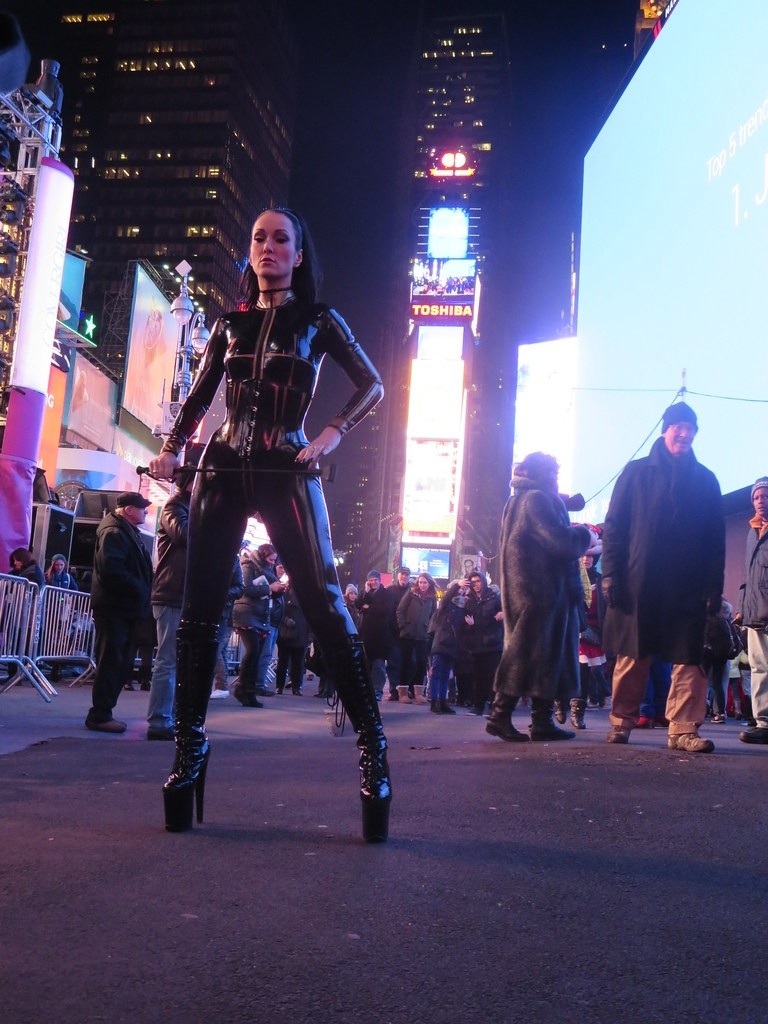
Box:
[738,727,768,744]
[124,682,151,691]
[209,690,230,699]
[636,715,669,729]
[257,675,334,698]
[375,685,494,717]
[711,714,727,723]
[85,718,127,733]
[233,682,264,708]
[147,725,176,741]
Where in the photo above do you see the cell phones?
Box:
[467,579,475,587]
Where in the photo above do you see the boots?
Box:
[485,692,530,742]
[320,635,393,844]
[162,620,221,833]
[553,698,587,729]
[530,701,576,741]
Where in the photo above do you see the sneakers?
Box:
[606,726,631,744]
[668,733,715,753]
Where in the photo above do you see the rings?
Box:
[153,470,158,473]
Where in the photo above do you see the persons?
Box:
[0,400,768,753]
[413,272,475,294]
[148,205,391,842]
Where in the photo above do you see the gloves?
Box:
[705,591,722,614]
[601,575,624,609]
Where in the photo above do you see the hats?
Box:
[751,477,768,503]
[662,401,698,432]
[397,567,411,575]
[346,584,359,596]
[116,492,152,508]
[173,466,196,490]
